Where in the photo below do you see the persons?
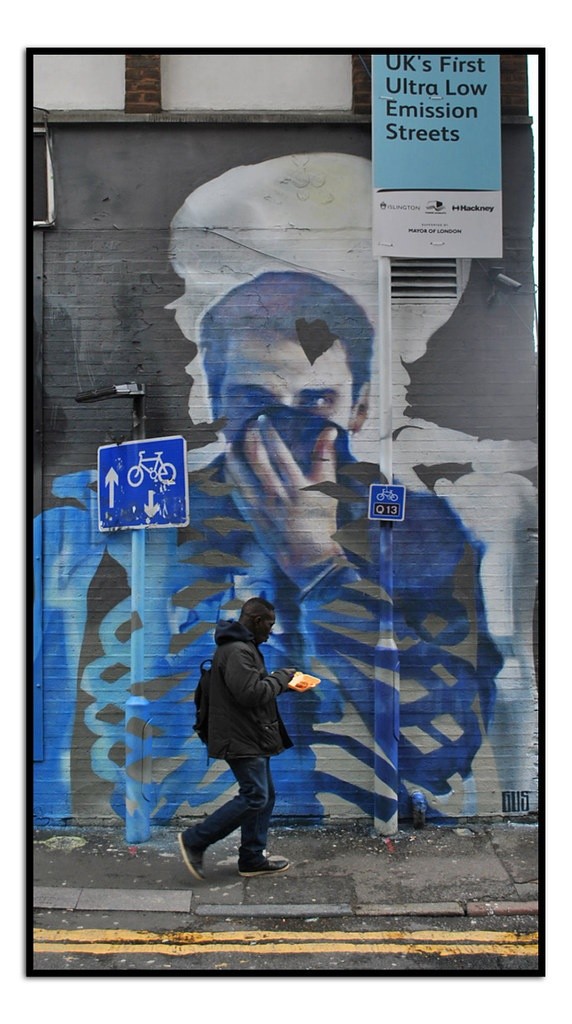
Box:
[178,597,296,879]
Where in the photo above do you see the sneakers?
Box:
[178,832,206,881]
[237,855,290,876]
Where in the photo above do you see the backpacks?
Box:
[192,658,212,744]
